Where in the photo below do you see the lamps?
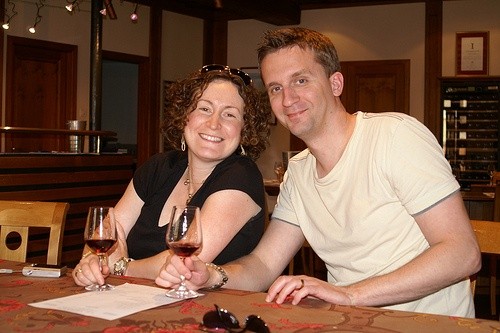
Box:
[130,2,139,20]
[29,25,37,33]
[2,20,10,30]
[65,0,77,12]
[99,1,113,15]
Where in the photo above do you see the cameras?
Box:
[21,263,68,278]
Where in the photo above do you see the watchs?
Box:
[113,256,135,278]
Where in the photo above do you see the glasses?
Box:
[199,303,269,333]
[199,64,252,88]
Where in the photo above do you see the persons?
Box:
[155,25,481,320]
[71,65,271,288]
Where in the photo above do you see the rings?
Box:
[81,262,89,268]
[75,268,82,277]
[296,278,305,292]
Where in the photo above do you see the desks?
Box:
[0,258,500,333]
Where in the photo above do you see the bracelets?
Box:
[82,251,107,264]
[204,263,230,290]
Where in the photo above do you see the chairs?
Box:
[0,200,68,266]
[266,193,316,278]
[468,217,500,319]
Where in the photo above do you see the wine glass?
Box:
[83,207,118,292]
[165,204,203,299]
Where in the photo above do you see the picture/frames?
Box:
[455,31,489,77]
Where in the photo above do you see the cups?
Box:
[68,120,87,154]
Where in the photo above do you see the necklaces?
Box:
[184,163,216,205]
